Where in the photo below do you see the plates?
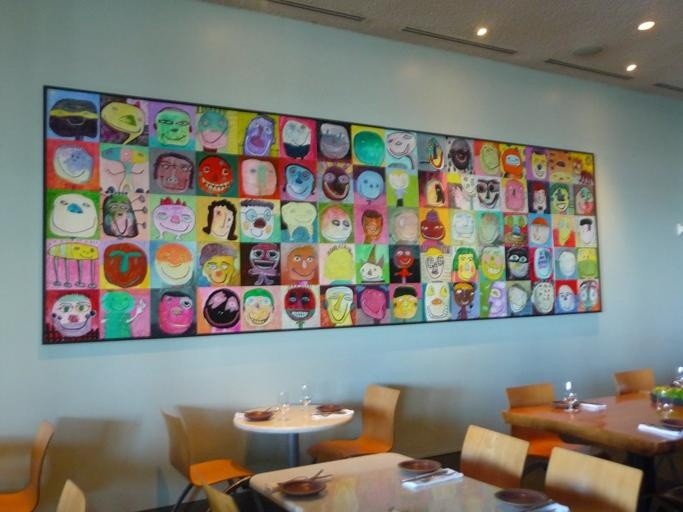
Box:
[397,459,442,474]
[316,404,343,412]
[493,488,549,508]
[660,418,683,431]
[245,411,275,421]
[281,479,324,496]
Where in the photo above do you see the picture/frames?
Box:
[39,82,604,346]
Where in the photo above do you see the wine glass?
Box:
[656,389,674,419]
[276,391,290,422]
[561,380,581,414]
[297,384,312,411]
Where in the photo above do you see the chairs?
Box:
[460,425,530,488]
[0,419,88,511]
[306,383,402,463]
[546,445,643,512]
[165,414,257,512]
[236,429,254,479]
[611,367,657,397]
[504,382,582,477]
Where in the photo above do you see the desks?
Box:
[250,452,566,510]
[235,406,356,465]
